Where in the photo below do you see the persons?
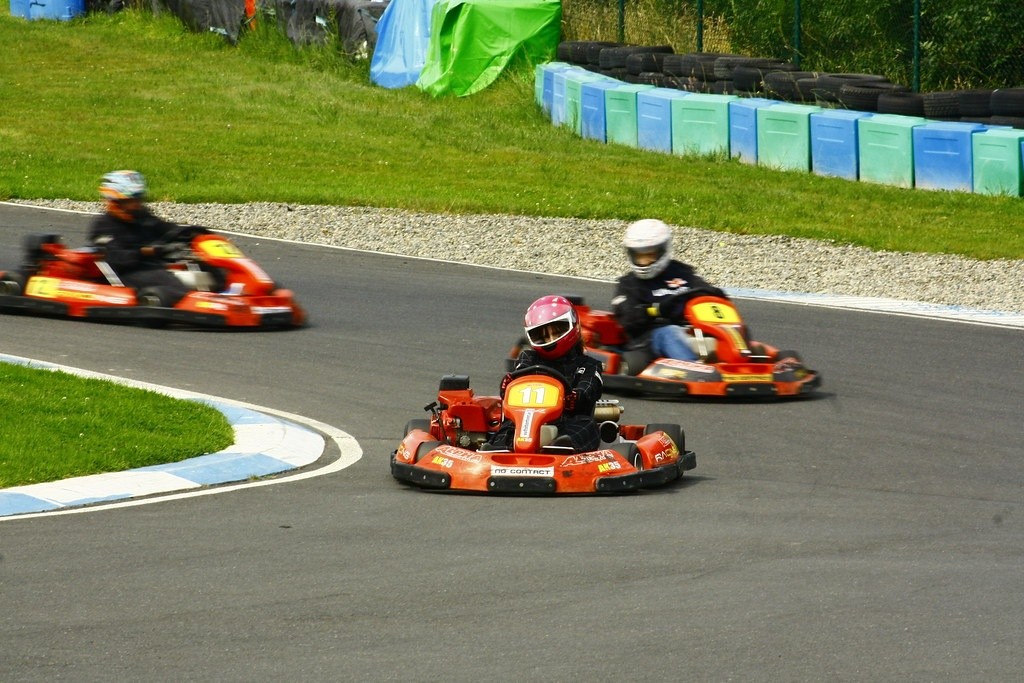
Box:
[480,296,606,452]
[83,167,213,292]
[611,217,768,364]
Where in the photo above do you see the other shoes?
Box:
[482,443,492,451]
[550,434,572,453]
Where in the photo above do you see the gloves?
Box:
[659,296,684,319]
[570,389,594,415]
[501,373,513,389]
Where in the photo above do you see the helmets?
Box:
[623,219,672,280]
[524,295,581,361]
[99,170,144,221]
